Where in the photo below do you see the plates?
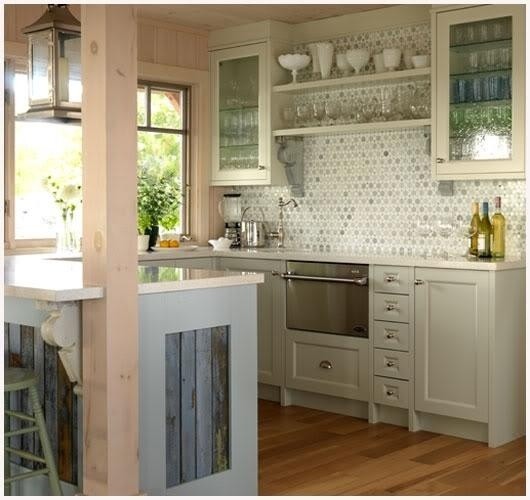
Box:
[150,245,198,251]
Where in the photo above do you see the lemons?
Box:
[160,240,168,247]
[170,240,179,247]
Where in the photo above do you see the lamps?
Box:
[15,4,82,124]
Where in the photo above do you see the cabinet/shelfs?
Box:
[273,67,431,137]
[429,4,526,181]
[207,20,293,187]
[286,330,370,403]
[219,259,287,407]
[372,266,489,426]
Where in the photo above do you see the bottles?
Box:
[468,196,505,260]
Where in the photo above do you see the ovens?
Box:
[280,261,369,339]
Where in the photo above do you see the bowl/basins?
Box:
[208,237,234,248]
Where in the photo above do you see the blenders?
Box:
[217,190,241,247]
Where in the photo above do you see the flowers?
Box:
[41,173,83,223]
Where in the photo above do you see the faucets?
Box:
[270,197,298,247]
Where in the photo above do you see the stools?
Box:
[4,366,63,496]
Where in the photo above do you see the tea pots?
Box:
[241,206,268,248]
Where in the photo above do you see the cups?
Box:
[138,235,150,251]
[383,47,402,71]
[411,56,428,68]
[450,16,512,103]
[372,54,387,73]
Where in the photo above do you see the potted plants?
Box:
[137,207,153,251]
[137,165,191,247]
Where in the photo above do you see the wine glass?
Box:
[281,86,431,129]
[219,76,259,169]
[335,49,371,79]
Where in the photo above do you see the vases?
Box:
[56,214,80,255]
